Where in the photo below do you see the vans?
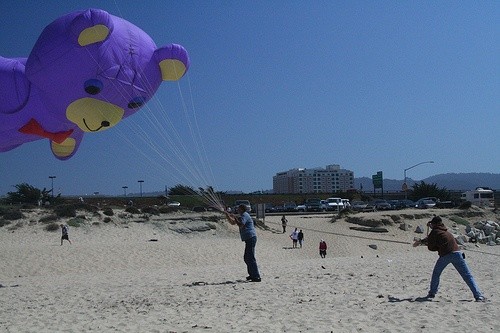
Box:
[305,198,351,211]
[460,193,466,202]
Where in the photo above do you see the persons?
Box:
[282,215,288,233]
[224,205,262,282]
[289,228,304,247]
[59,223,72,246]
[422,216,490,303]
[319,240,327,258]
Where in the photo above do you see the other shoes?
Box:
[246,276,251,280]
[423,295,434,300]
[249,277,261,282]
[476,296,491,302]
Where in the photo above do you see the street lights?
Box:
[122,186,128,195]
[404,161,434,203]
[48,176,56,197]
[138,181,144,196]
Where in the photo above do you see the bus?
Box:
[466,188,495,206]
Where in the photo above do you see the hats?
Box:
[238,204,247,210]
[428,216,442,224]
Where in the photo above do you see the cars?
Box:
[192,207,207,212]
[350,198,437,211]
[168,201,180,207]
[251,204,306,213]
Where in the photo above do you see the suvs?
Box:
[233,200,251,214]
[253,190,261,196]
[208,199,226,214]
